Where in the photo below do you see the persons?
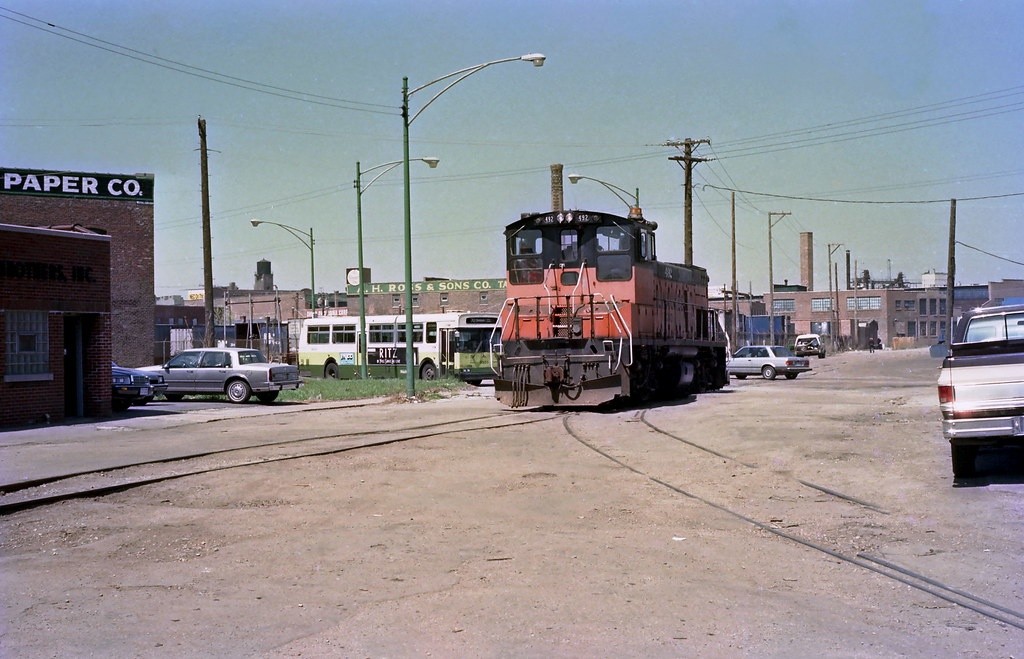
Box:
[869,338,875,353]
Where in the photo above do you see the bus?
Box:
[298,312,503,384]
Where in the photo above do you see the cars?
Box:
[726,346,812,380]
[134,347,303,403]
[112,361,169,410]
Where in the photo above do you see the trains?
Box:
[490,209,728,407]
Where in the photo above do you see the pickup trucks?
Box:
[929,304,1024,476]
[794,334,826,358]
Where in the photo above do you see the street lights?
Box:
[400,52,545,399]
[568,174,639,208]
[353,158,440,379]
[251,220,316,318]
